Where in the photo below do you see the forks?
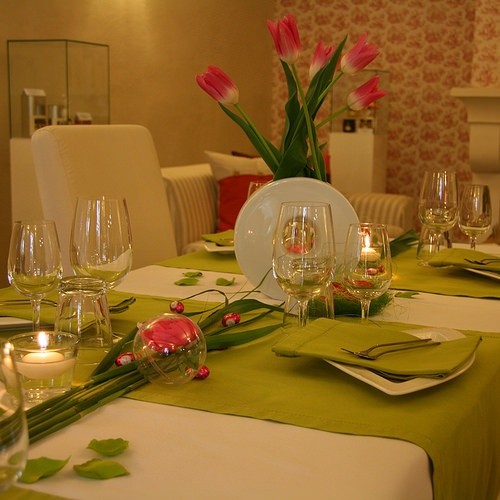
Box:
[341,341,441,361]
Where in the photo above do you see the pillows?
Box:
[206,150,275,234]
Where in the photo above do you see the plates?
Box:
[204,240,236,256]
[0,317,34,330]
[453,264,500,281]
[321,328,476,396]
[387,224,411,243]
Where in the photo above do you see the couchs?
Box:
[164,164,419,255]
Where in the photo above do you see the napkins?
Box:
[198,229,235,245]
[269,317,484,380]
[426,244,500,273]
[0,275,136,335]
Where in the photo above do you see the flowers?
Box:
[195,12,391,179]
[139,315,201,374]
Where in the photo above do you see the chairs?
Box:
[31,122,176,271]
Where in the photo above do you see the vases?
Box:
[232,175,362,304]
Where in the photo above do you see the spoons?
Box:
[0,296,136,314]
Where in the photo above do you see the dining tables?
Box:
[0,233,500,500]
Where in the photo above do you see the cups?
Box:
[281,259,335,324]
[351,233,384,277]
[1,338,29,492]
[6,330,78,407]
[53,276,113,366]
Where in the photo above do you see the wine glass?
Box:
[457,183,492,250]
[7,220,63,330]
[418,170,457,268]
[272,200,336,357]
[342,223,391,320]
[71,196,133,343]
